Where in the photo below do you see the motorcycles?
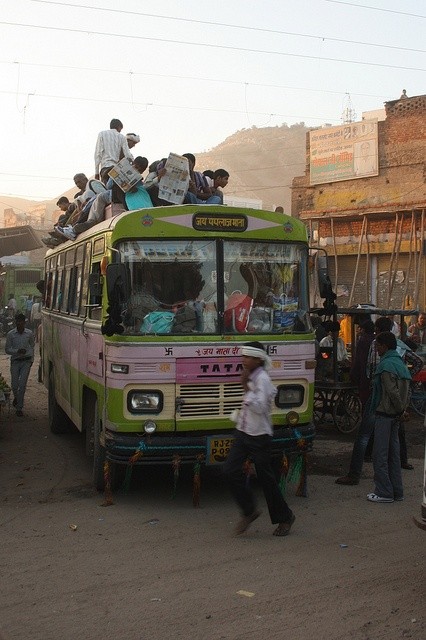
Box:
[0,305,30,336]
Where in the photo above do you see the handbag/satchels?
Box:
[247,306,274,334]
[225,290,253,333]
[125,183,153,210]
[139,311,176,333]
[175,300,197,333]
[272,293,298,333]
[199,300,218,334]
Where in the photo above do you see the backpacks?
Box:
[123,305,151,328]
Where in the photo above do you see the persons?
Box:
[25,295,33,317]
[4,314,35,417]
[319,320,349,362]
[39,117,231,249]
[349,313,376,386]
[367,316,425,470]
[408,312,426,345]
[366,331,413,503]
[215,341,296,537]
[6,294,16,312]
[30,298,42,341]
[36,279,47,294]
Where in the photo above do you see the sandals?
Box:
[237,509,262,533]
[273,509,295,536]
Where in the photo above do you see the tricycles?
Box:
[313,350,426,434]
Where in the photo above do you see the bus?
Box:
[39,203,332,493]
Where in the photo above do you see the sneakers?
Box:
[401,464,414,469]
[41,237,56,249]
[12,400,19,407]
[395,497,404,501]
[16,410,24,417]
[48,231,69,243]
[367,493,394,503]
[336,476,360,485]
[55,224,78,241]
